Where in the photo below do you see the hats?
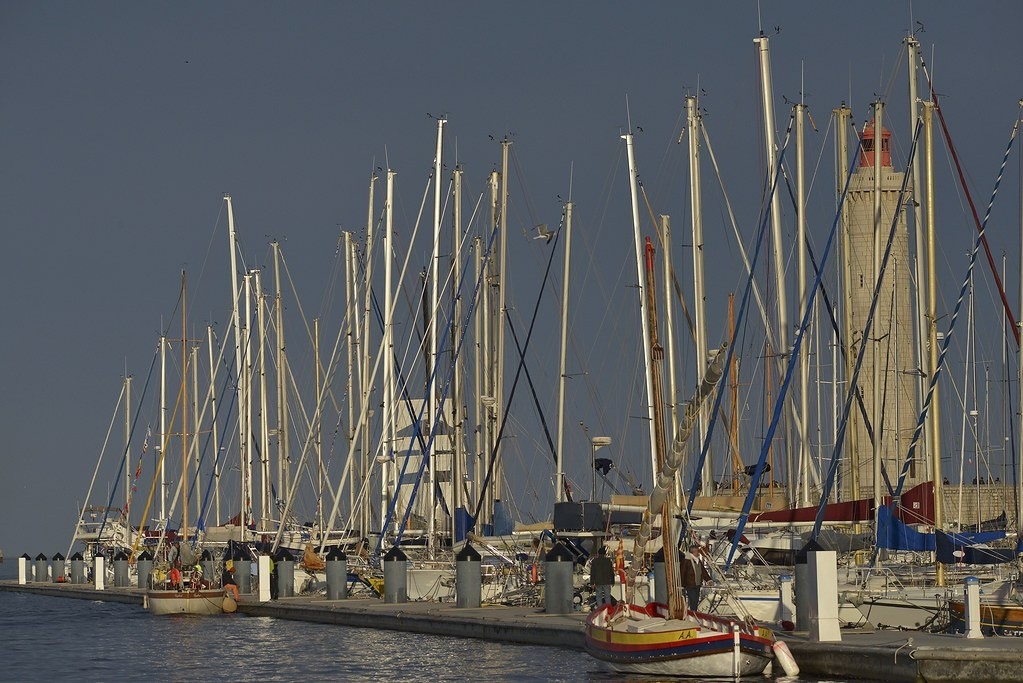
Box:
[598,545,606,555]
[230,567,236,573]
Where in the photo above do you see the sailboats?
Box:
[58,33,1023,638]
[575,233,776,680]
[139,268,239,617]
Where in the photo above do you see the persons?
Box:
[591,547,614,606]
[680,545,712,610]
[973,476,985,484]
[943,477,949,484]
[987,478,1000,484]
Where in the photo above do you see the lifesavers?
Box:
[773,640,800,677]
[531,564,537,584]
[171,568,180,586]
